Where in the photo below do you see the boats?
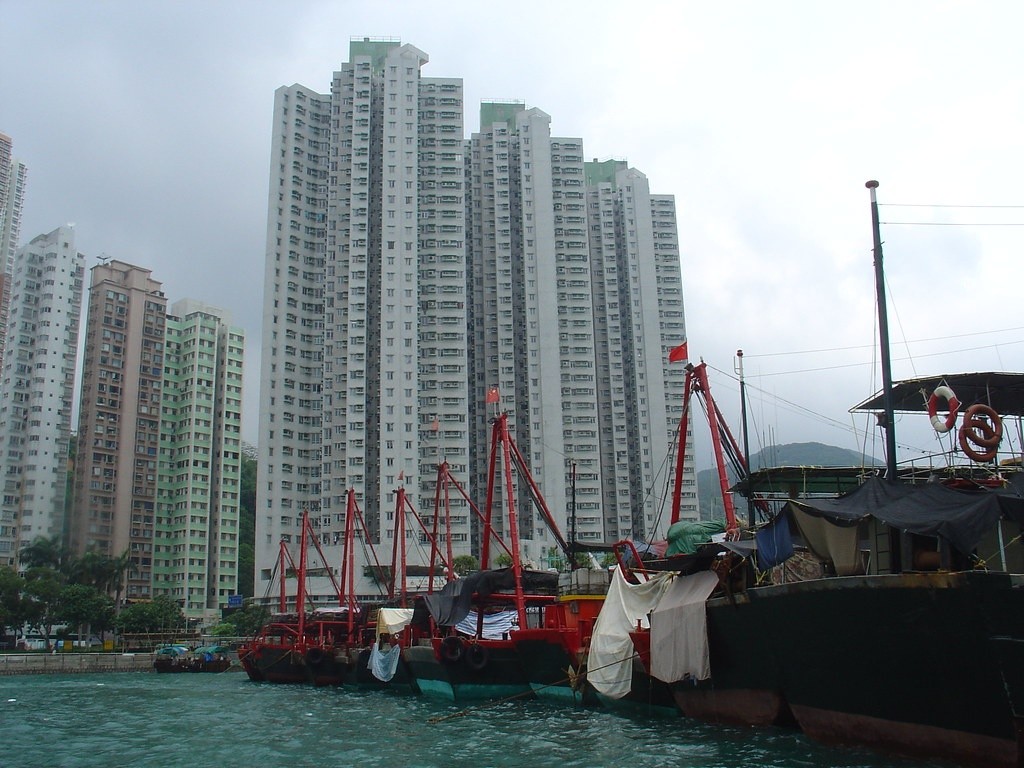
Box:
[156,643,234,674]
[241,182,1024,768]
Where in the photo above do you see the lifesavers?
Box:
[244,649,255,661]
[928,386,959,434]
[725,528,739,542]
[306,647,324,666]
[958,403,1004,463]
[440,636,465,663]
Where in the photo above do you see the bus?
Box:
[17,634,58,652]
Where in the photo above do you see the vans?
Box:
[58,633,93,649]
